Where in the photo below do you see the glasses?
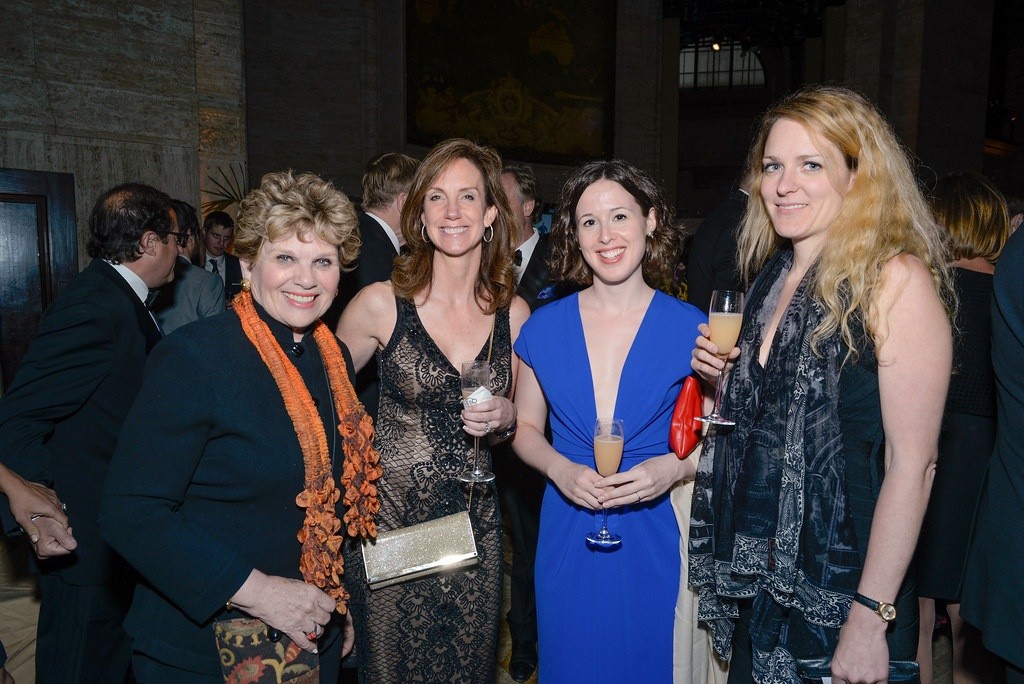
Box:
[147,228,189,249]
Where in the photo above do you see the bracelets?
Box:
[26,512,49,520]
[225,597,235,608]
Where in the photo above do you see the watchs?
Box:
[854,592,896,623]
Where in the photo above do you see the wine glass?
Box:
[456,359,495,483]
[587,417,625,546]
[693,290,746,426]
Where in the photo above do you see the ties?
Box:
[208,259,219,276]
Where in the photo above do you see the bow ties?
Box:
[144,290,160,310]
[509,249,525,267]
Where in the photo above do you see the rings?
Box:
[307,620,318,636]
[485,421,491,434]
[635,492,641,502]
[59,503,67,512]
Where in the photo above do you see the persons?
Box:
[0,183,182,684]
[673,166,766,316]
[321,151,423,430]
[910,164,1011,684]
[105,169,383,684]
[334,138,530,684]
[490,162,592,684]
[957,217,1024,684]
[509,159,708,684]
[202,211,244,312]
[998,189,1024,238]
[143,199,225,336]
[690,86,956,684]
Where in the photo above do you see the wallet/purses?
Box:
[668,376,703,460]
[796,655,920,684]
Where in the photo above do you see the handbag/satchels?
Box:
[360,510,479,591]
[213,619,321,684]
